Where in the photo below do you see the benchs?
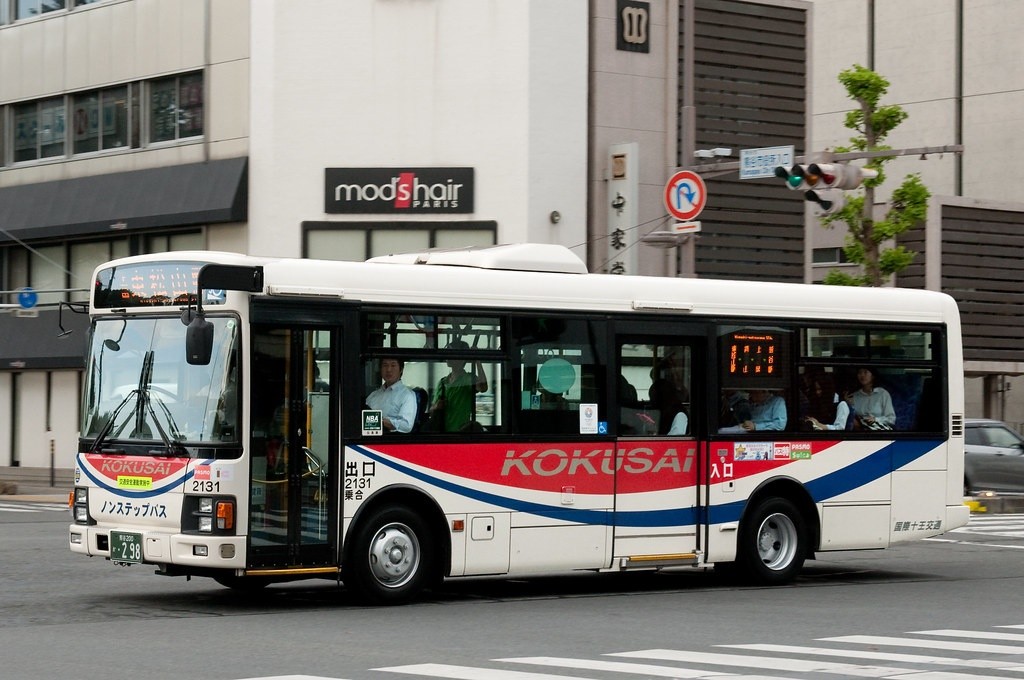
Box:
[876,372,925,431]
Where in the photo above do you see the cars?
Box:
[964,418,1024,496]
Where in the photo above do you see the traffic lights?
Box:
[774,163,861,191]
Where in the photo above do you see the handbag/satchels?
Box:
[419,377,445,432]
[855,415,893,430]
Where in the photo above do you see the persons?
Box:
[186,354,237,436]
[530,350,901,433]
[429,341,488,431]
[366,358,417,433]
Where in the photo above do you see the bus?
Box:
[57,242,970,607]
